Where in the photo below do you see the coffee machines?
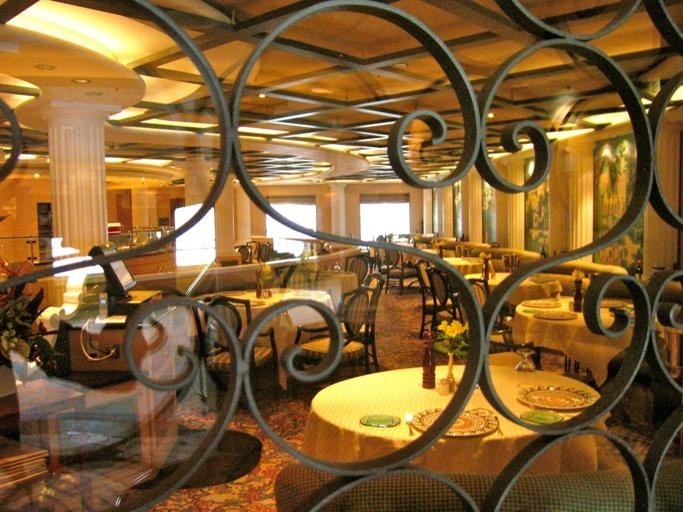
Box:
[234,245,251,265]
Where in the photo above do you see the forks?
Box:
[405,414,412,437]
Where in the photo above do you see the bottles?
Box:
[263,289,272,298]
[437,245,442,259]
[573,275,582,312]
[421,330,437,387]
[633,260,642,282]
[413,239,415,248]
[481,258,489,281]
[439,379,448,396]
[540,246,545,259]
[460,233,468,241]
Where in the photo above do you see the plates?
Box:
[411,408,498,437]
[516,385,600,410]
[522,301,561,308]
[532,310,576,320]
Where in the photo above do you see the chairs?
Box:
[0,225,683,511]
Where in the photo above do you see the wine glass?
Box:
[514,349,534,373]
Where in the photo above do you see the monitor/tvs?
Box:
[87,240,137,299]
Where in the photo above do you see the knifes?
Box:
[494,416,503,436]
